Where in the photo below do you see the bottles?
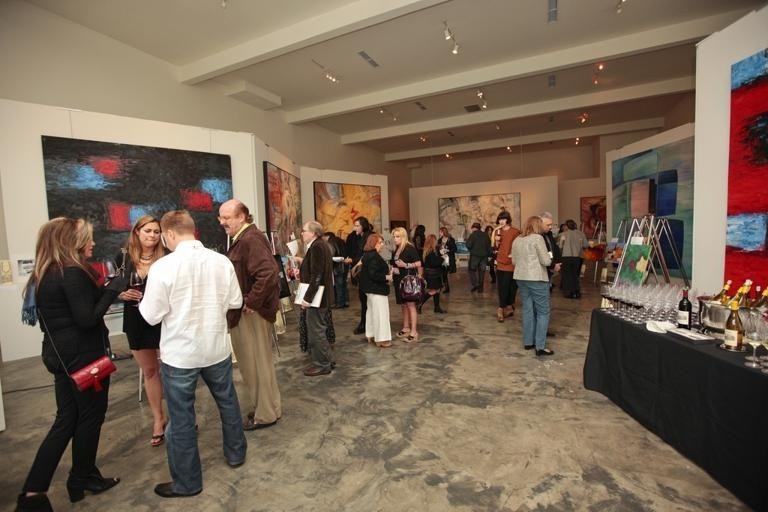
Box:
[725,301,744,352]
[712,279,768,308]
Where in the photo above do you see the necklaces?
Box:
[138,255,154,265]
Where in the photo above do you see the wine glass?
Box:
[104,256,119,286]
[601,281,709,328]
[745,317,768,374]
[131,271,145,306]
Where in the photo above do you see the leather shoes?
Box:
[304,365,332,376]
[535,348,555,357]
[344,303,349,307]
[525,344,537,350]
[308,361,337,367]
[545,333,555,338]
[354,325,365,335]
[228,457,243,469]
[330,303,346,309]
[443,289,449,293]
[153,481,203,497]
[471,284,481,293]
[491,279,496,284]
[243,415,277,432]
[247,411,282,420]
[478,285,483,293]
[566,293,581,299]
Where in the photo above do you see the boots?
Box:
[416,293,432,314]
[434,292,449,313]
[497,307,505,322]
[504,304,514,316]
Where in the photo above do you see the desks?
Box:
[583,307,767,512]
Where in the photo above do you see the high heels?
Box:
[14,490,54,512]
[366,336,374,343]
[66,464,122,504]
[375,340,392,348]
[396,329,411,338]
[150,421,166,446]
[404,331,419,342]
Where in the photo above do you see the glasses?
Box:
[301,229,313,233]
[218,214,242,222]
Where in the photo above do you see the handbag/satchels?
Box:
[67,354,118,393]
[399,274,426,301]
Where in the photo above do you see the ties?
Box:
[543,233,551,251]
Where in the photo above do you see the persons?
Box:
[138,209,247,498]
[110,216,198,448]
[12,216,129,512]
[218,199,282,431]
[295,216,456,377]
[466,212,588,355]
